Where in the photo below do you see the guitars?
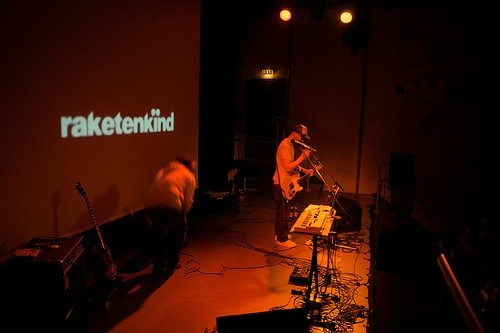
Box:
[281,164,323,201]
[74,181,117,283]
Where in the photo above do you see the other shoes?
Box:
[275,235,297,248]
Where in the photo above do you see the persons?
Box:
[143,154,195,277]
[272,124,314,248]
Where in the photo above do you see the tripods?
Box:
[300,234,337,328]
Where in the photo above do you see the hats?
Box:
[290,124,310,139]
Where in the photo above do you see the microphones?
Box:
[290,139,316,152]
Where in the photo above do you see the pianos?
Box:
[288,203,337,298]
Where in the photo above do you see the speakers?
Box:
[327,195,362,233]
[215,308,310,333]
